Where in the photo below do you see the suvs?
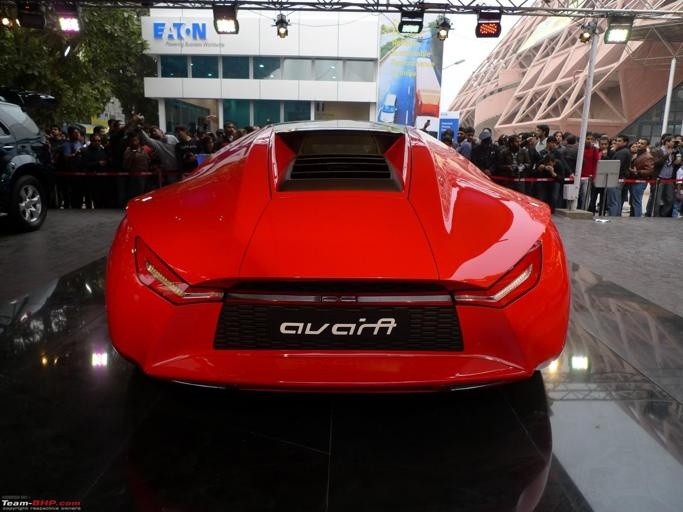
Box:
[1,99,56,231]
[378,93,397,123]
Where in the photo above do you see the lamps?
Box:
[1,0,683,52]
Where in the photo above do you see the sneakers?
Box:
[46,200,125,210]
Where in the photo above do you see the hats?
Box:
[599,134,610,142]
[478,127,492,141]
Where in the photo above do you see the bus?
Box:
[412,55,439,133]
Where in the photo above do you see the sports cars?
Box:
[104,117,571,398]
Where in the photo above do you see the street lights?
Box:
[441,58,465,71]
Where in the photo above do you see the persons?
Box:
[440,124,683,218]
[44,112,261,210]
